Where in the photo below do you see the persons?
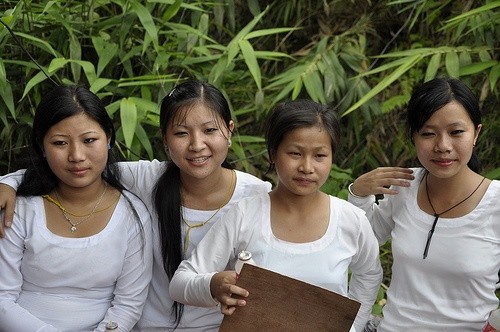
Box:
[347,77,500,332]
[0,78,273,332]
[169,100,383,332]
[0,85,152,332]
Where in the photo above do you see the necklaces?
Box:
[180,169,234,259]
[42,180,121,233]
[422,171,486,261]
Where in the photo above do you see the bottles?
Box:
[235,251,256,281]
[482,308,500,332]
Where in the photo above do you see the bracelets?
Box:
[348,183,369,197]
